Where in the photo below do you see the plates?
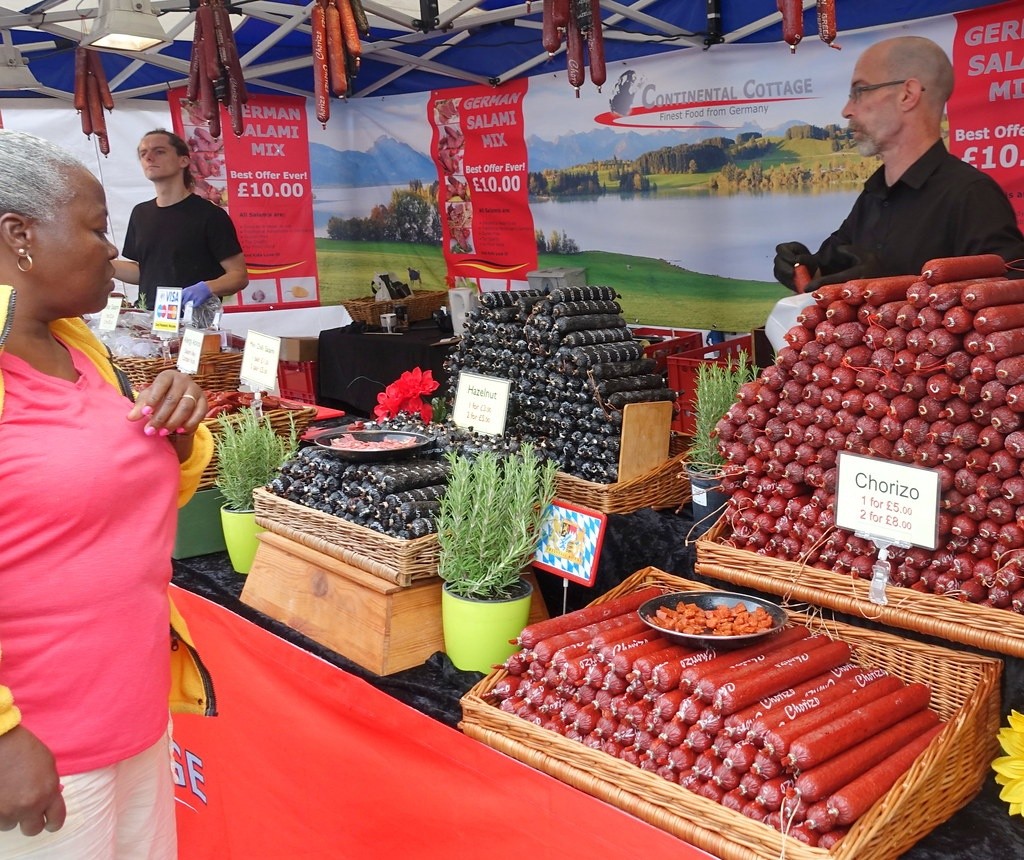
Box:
[314,430,431,462]
[638,590,789,650]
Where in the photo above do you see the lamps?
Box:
[0,26,43,93]
[79,1,171,52]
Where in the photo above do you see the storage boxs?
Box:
[627,318,775,439]
[276,334,322,406]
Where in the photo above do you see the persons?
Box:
[109,130,249,318]
[774,37,1024,294]
[0,129,206,860]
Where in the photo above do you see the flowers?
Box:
[372,368,445,423]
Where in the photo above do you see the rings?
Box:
[181,395,197,406]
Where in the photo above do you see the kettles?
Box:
[448,287,475,336]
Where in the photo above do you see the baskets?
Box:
[695,504,1024,657]
[553,430,698,514]
[458,566,1003,860]
[249,484,542,587]
[341,289,448,325]
[113,350,318,492]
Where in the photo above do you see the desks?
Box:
[320,317,465,417]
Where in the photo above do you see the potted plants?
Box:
[678,352,772,530]
[215,406,305,577]
[431,448,562,676]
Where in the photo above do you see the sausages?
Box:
[706,254,1024,620]
[487,586,948,850]
[265,445,457,542]
[374,285,686,486]
[542,0,607,98]
[186,2,247,137]
[200,389,309,419]
[310,0,371,130]
[776,0,842,51]
[73,44,113,156]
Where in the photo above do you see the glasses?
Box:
[849,80,926,103]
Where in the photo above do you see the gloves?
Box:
[804,246,902,293]
[182,281,214,309]
[773,241,812,293]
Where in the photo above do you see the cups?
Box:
[394,305,409,332]
[380,314,397,334]
